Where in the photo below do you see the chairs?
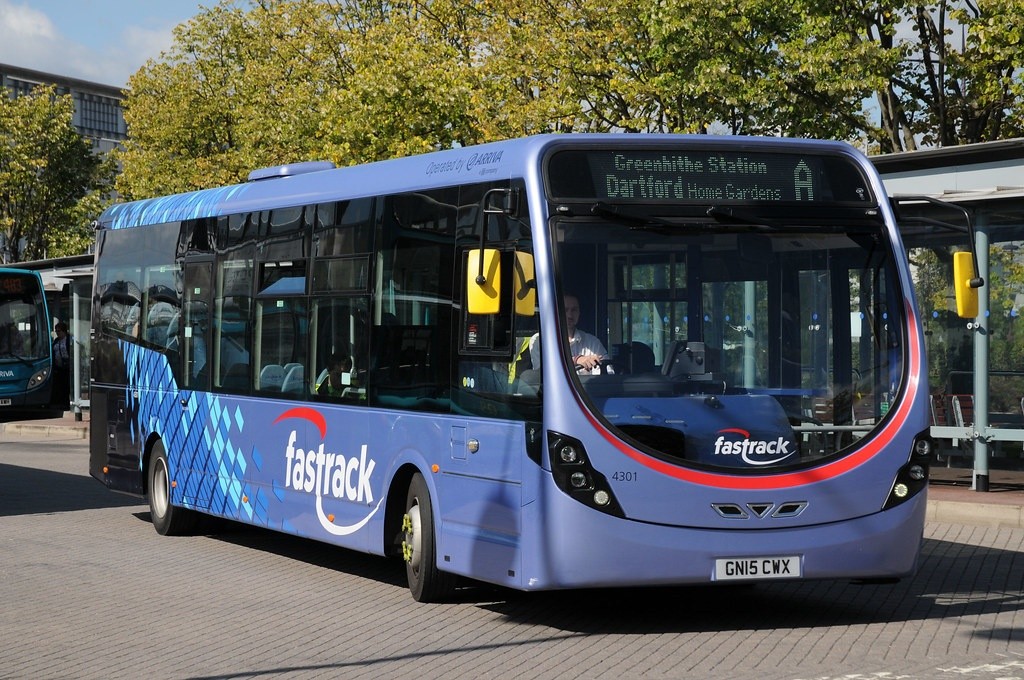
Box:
[615,342,655,374]
[126,322,332,392]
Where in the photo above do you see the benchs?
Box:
[807,392,1001,468]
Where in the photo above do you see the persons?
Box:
[0,326,24,354]
[528,295,615,375]
[49,322,70,402]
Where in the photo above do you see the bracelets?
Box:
[574,355,582,365]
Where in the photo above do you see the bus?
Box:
[0,268,59,407]
[87,135,987,602]
